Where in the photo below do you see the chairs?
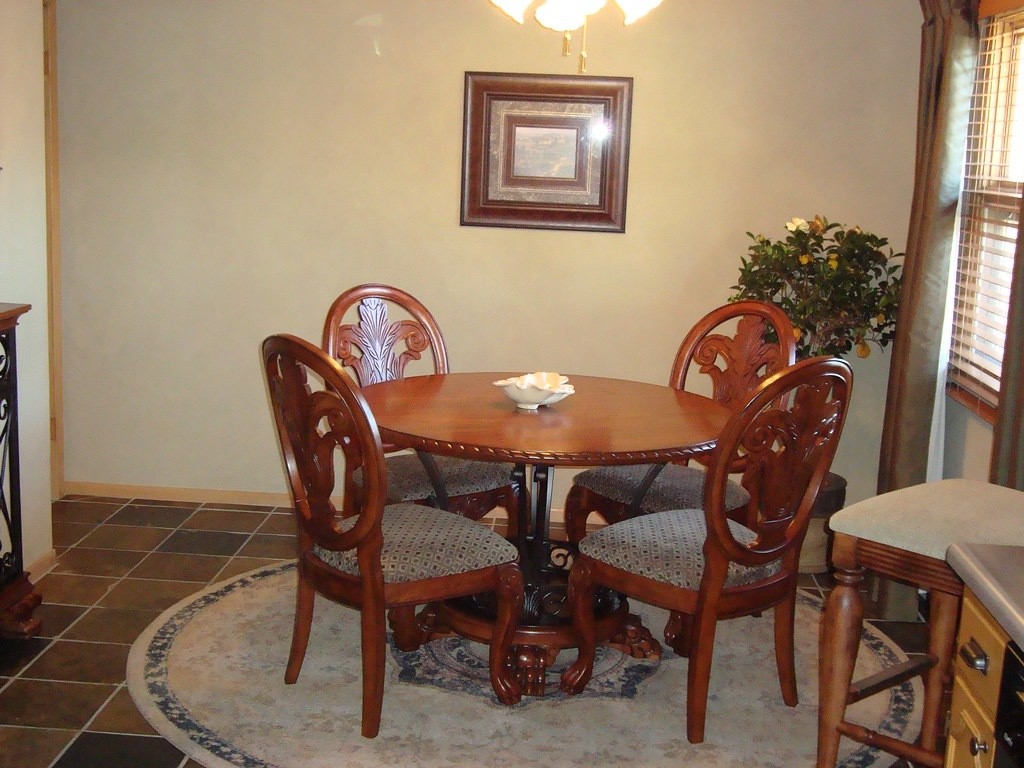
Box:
[261,283,854,745]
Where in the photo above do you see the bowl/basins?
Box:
[493,372,576,409]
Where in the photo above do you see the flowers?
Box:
[728,215,907,361]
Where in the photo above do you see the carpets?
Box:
[126,556,926,768]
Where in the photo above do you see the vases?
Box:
[753,472,847,618]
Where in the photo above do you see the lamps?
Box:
[489,0,662,72]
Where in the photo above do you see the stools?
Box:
[817,477,1024,768]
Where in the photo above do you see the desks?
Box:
[358,371,740,697]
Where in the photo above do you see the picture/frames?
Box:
[459,71,633,233]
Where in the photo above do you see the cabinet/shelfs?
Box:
[944,583,1024,768]
